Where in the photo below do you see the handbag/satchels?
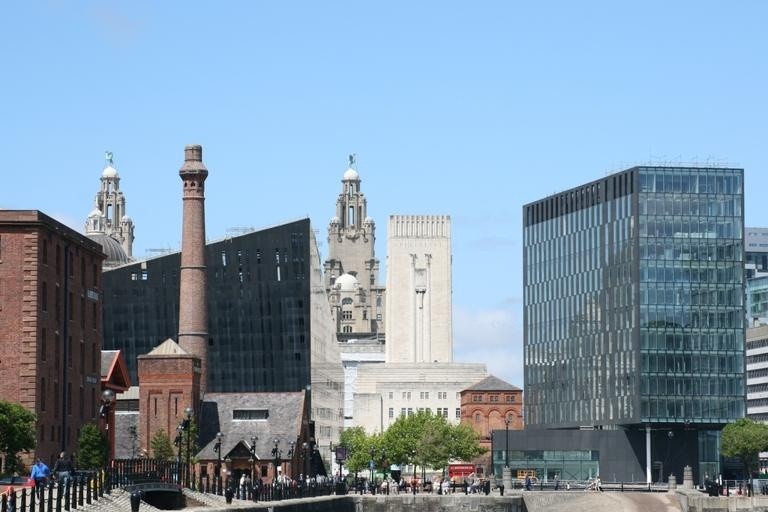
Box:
[27,478,35,486]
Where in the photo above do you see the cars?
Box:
[0,469,95,487]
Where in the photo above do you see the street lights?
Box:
[184,407,194,488]
[502,418,511,489]
[272,437,280,498]
[289,440,295,497]
[353,443,417,494]
[314,444,318,490]
[667,430,676,492]
[301,442,308,495]
[176,423,184,487]
[101,389,114,490]
[488,428,497,488]
[251,435,258,500]
[683,418,694,488]
[216,431,224,495]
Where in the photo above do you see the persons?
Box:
[524,474,533,490]
[53,451,74,497]
[596,475,604,492]
[553,472,560,490]
[31,457,52,499]
[238,471,485,500]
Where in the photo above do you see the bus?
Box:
[449,463,475,477]
